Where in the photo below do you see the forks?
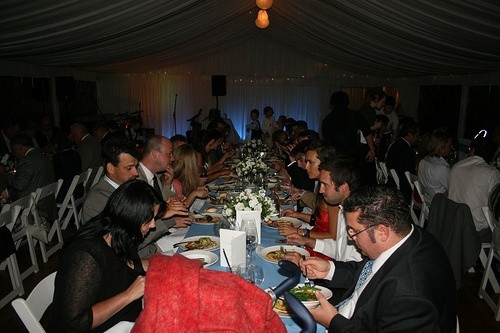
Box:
[302,254,312,287]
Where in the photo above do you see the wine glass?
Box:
[240,217,259,262]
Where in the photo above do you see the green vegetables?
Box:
[289,285,322,300]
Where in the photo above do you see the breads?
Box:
[206,207,217,212]
[219,192,227,196]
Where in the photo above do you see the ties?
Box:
[335,260,373,308]
[153,174,163,199]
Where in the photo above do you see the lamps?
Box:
[255,0,274,28]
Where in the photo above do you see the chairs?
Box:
[0,149,500,333]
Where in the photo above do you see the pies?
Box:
[274,298,293,314]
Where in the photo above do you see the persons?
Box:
[299,184,454,333]
[0,89,500,333]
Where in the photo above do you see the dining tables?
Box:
[140,143,329,333]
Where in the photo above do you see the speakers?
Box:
[56,76,74,101]
[212,75,226,96]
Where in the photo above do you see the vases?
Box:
[244,152,268,160]
[235,210,261,245]
[242,172,265,192]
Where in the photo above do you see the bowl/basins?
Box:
[280,283,333,308]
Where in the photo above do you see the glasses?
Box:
[346,223,389,240]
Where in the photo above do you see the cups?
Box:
[226,263,265,286]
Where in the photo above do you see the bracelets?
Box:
[304,228,310,237]
[297,229,298,234]
[309,213,316,226]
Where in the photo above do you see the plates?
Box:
[179,249,218,267]
[191,212,225,225]
[259,245,310,264]
[264,287,299,318]
[209,196,225,209]
[180,235,221,251]
[262,216,303,229]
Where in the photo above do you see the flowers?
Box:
[235,154,271,177]
[222,186,276,224]
[235,138,271,156]
